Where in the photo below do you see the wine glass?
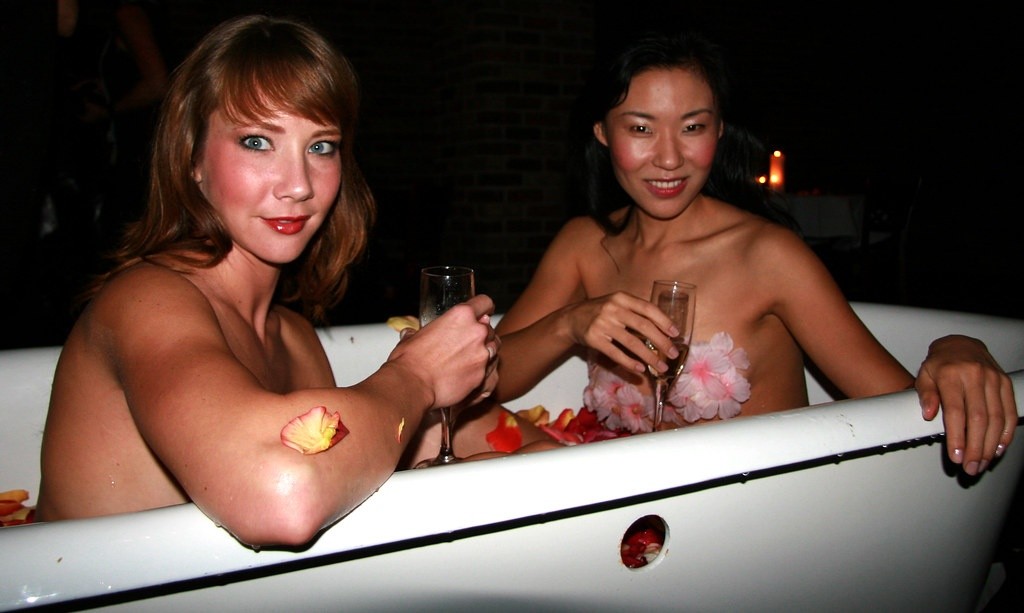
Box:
[645,280,697,432]
[413,266,474,469]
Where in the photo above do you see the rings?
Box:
[487,344,497,358]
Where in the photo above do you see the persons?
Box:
[1,0,170,352]
[449,33,1017,478]
[32,17,501,554]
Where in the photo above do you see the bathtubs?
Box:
[0,300,1024,613]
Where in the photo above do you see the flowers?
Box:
[582,330,754,433]
